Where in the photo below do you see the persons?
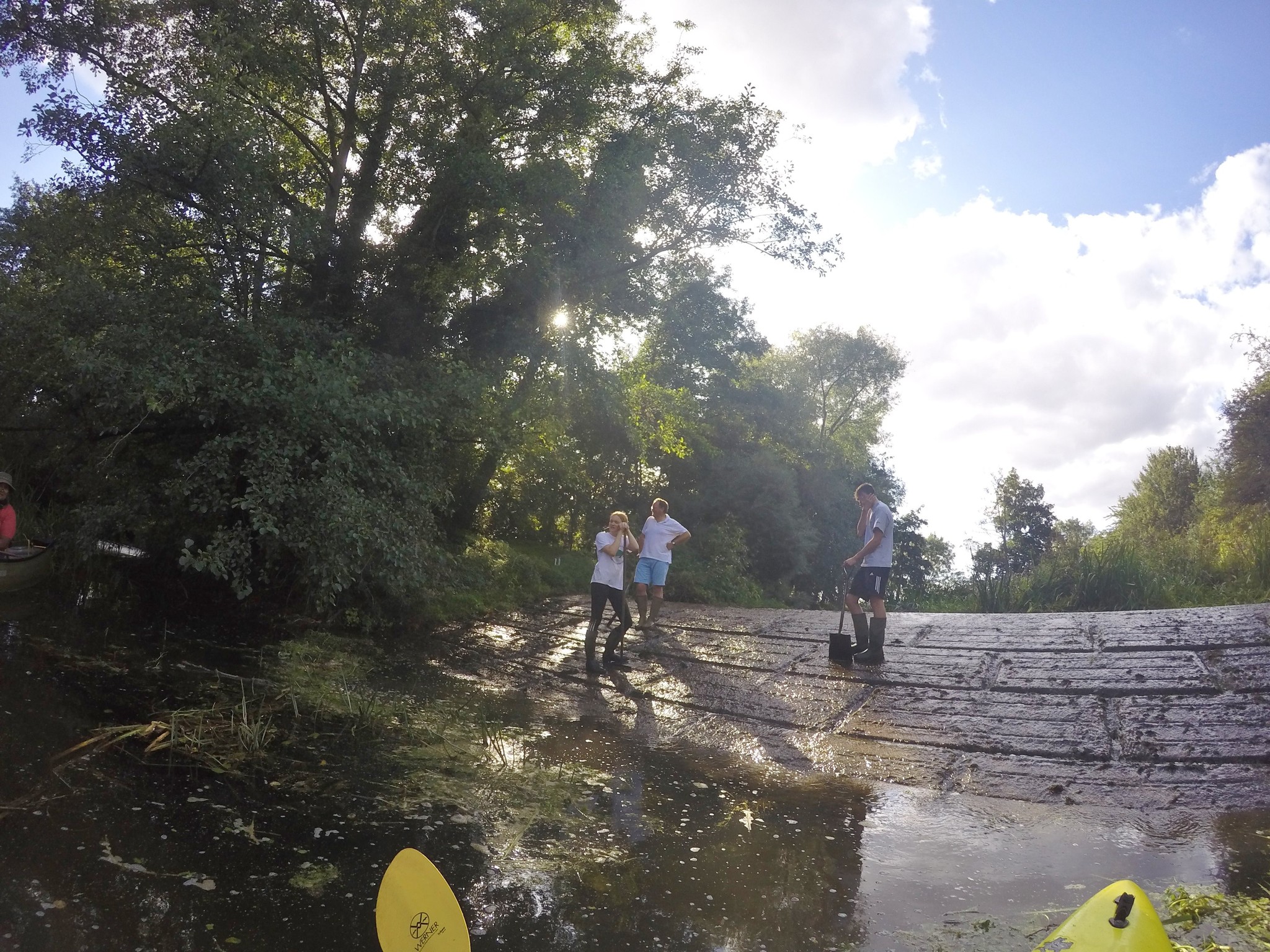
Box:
[584,510,639,674]
[633,498,692,631]
[840,483,894,663]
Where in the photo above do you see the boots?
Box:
[850,613,869,655]
[642,596,663,627]
[585,630,606,673]
[854,617,886,661]
[634,595,647,628]
[602,629,628,662]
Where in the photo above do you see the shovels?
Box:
[828,561,855,662]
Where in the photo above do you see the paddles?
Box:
[376,848,471,952]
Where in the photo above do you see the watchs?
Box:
[672,541,676,546]
